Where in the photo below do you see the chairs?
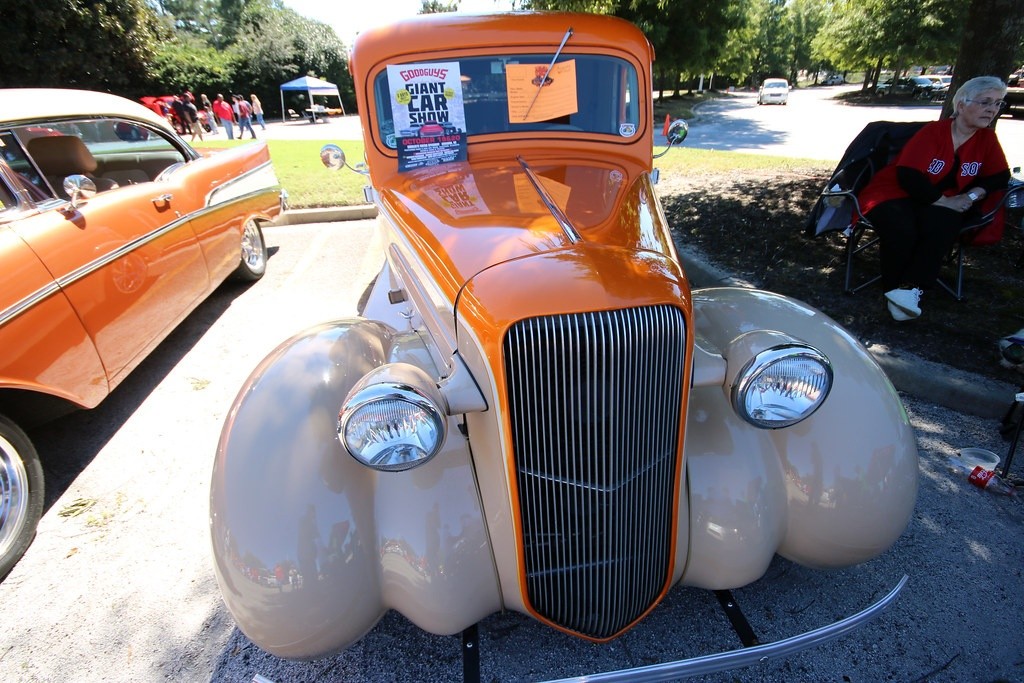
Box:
[1001,328,1024,480]
[26,136,183,199]
[288,109,320,123]
[818,121,1024,301]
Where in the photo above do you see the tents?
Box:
[280,76,346,123]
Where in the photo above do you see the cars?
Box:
[827,75,844,86]
[0,86,290,584]
[874,74,960,97]
[213,11,922,660]
[757,77,792,105]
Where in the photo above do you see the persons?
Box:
[157,89,267,142]
[298,93,318,123]
[858,76,1011,320]
[239,559,298,593]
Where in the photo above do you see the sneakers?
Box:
[885,287,923,316]
[887,301,916,321]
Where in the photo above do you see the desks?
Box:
[306,109,341,123]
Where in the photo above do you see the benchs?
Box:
[464,100,509,133]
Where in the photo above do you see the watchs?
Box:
[968,192,978,202]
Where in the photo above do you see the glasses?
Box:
[967,99,1006,110]
